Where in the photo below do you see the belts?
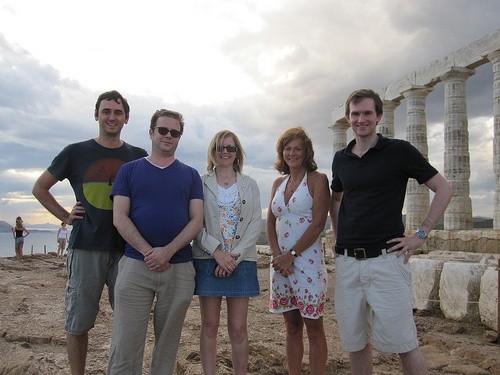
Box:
[335,243,403,260]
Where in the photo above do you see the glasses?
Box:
[216,146,237,152]
[158,127,182,138]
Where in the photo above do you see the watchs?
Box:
[416,229,428,239]
[290,248,298,257]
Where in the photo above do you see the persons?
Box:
[108,108,204,375]
[57,222,69,257]
[190,129,263,375]
[330,88,453,375]
[267,126,330,375]
[12,217,30,259]
[32,90,149,375]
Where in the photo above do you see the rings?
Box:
[228,266,230,269]
